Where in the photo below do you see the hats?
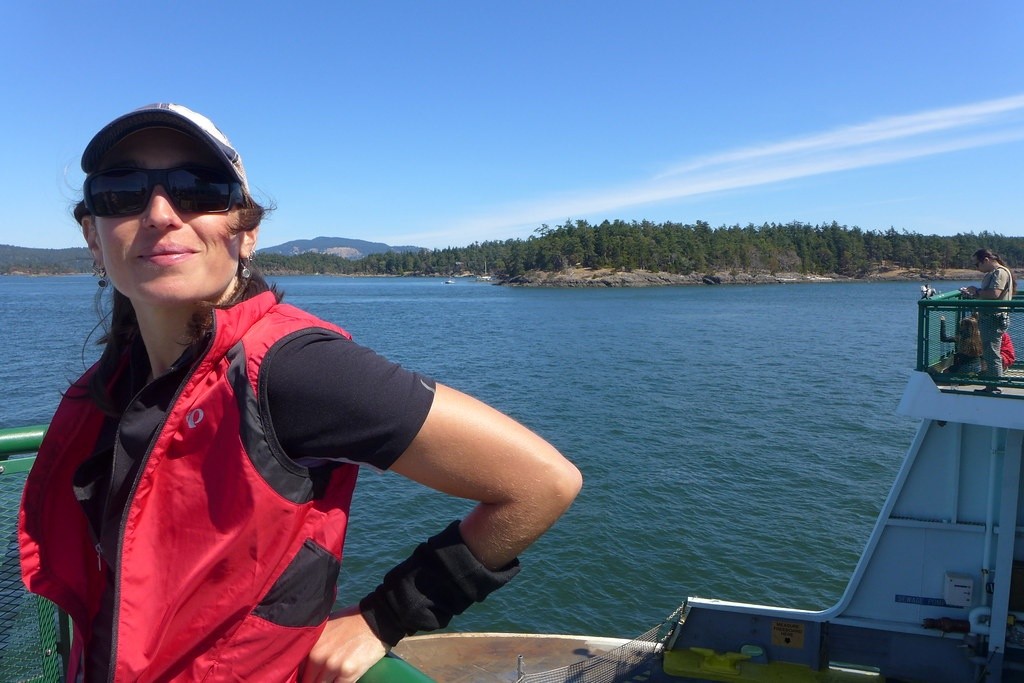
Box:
[81,102,250,196]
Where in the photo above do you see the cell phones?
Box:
[960,287,975,295]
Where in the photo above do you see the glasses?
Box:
[83,167,246,218]
[975,261,980,268]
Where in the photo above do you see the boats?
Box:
[0,287,1024,683]
[445,281,455,284]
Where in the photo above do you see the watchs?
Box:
[976,288,982,295]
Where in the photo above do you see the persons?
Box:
[937,315,983,381]
[16,97,583,683]
[971,312,1014,374]
[965,249,1015,394]
